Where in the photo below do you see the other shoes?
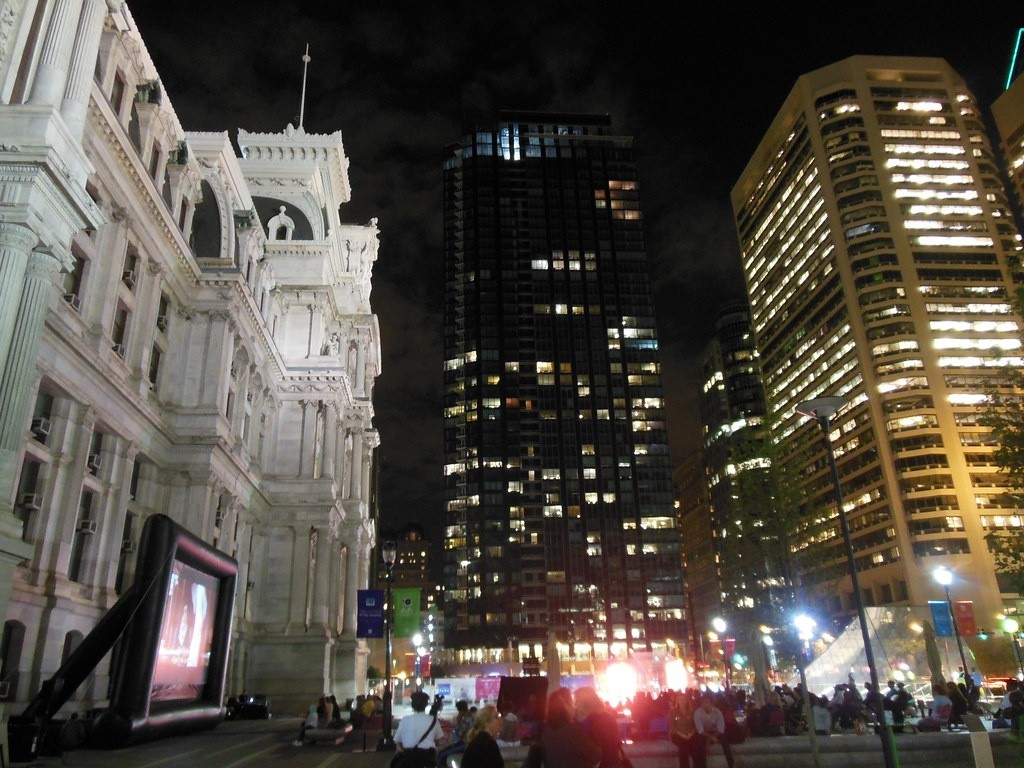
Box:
[292,741,303,749]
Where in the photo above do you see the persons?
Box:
[917,667,1024,732]
[350,694,382,729]
[325,335,339,356]
[638,673,917,768]
[390,686,634,768]
[292,695,340,746]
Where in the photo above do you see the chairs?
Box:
[790,704,807,734]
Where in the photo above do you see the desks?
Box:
[867,718,923,734]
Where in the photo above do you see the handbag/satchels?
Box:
[387,748,438,768]
[613,758,634,768]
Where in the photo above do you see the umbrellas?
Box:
[753,629,754,703]
[546,632,561,708]
[923,619,945,702]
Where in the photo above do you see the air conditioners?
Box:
[0,681,11,698]
[232,368,266,422]
[23,493,44,509]
[215,509,224,520]
[111,343,125,359]
[81,520,97,534]
[65,293,82,311]
[88,453,102,468]
[120,540,134,552]
[31,418,52,436]
[158,315,168,329]
[122,269,135,284]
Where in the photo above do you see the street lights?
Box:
[1004,616,1024,672]
[713,617,729,685]
[378,539,397,752]
[936,567,970,681]
[792,394,904,766]
